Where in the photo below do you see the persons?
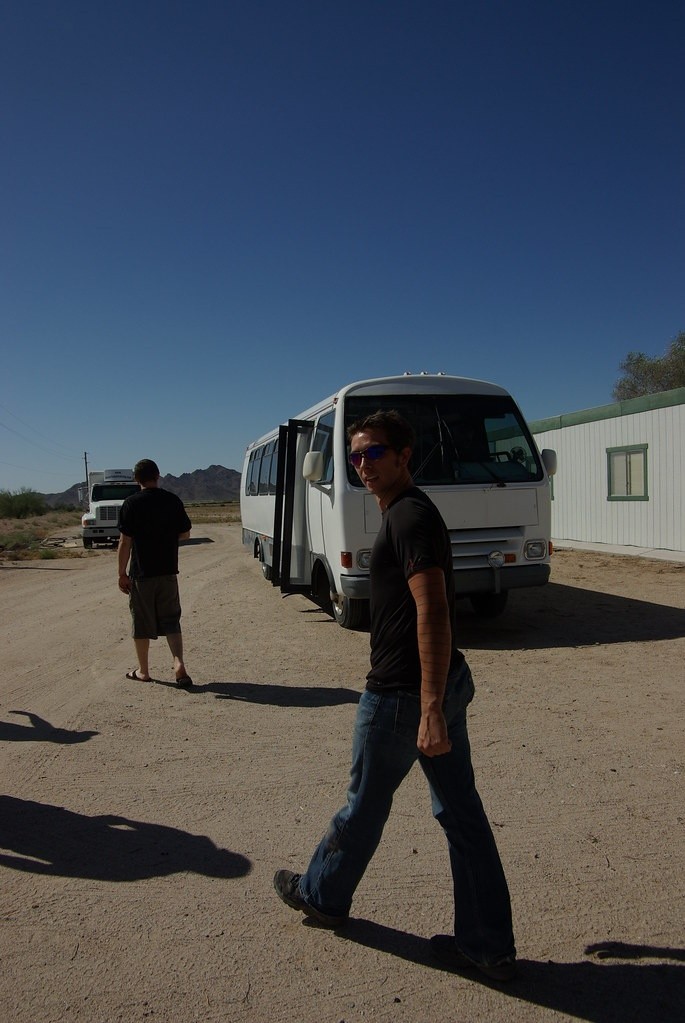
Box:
[275,409,518,976]
[118,459,193,690]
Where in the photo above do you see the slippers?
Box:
[176,672,192,689]
[126,669,151,682]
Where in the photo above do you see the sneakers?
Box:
[430,934,515,981]
[274,870,349,926]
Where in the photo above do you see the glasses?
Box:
[348,444,394,465]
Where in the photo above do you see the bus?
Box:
[240,370,560,629]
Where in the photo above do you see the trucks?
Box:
[81,469,142,549]
